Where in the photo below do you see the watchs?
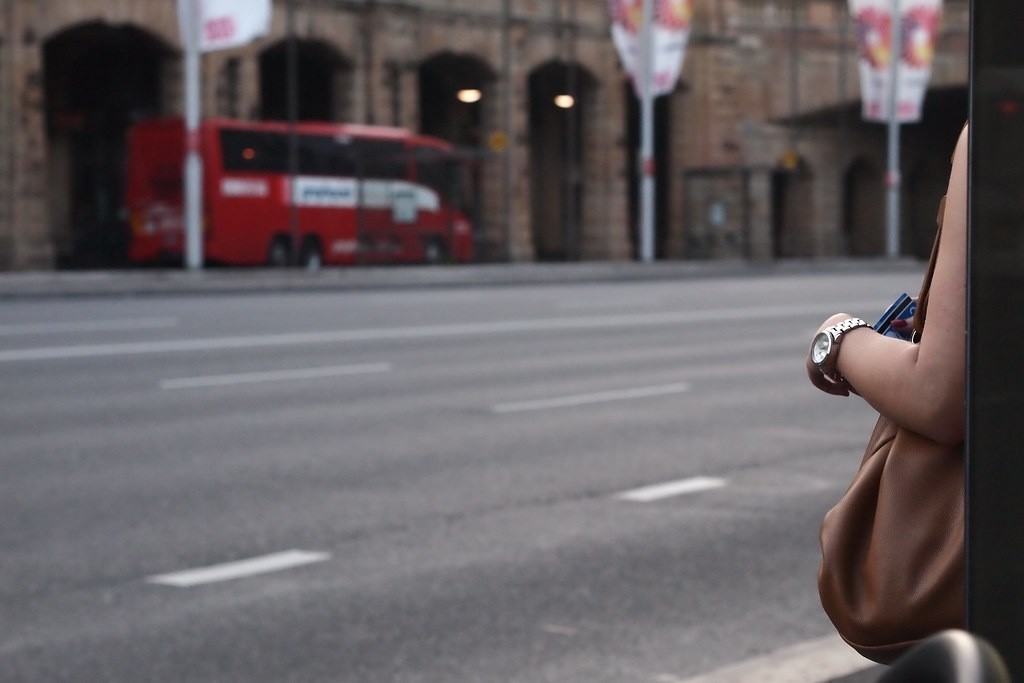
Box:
[815,317,874,389]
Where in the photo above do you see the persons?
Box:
[807,116,968,450]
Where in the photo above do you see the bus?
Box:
[127,117,475,271]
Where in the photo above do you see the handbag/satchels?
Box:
[815,414,969,665]
